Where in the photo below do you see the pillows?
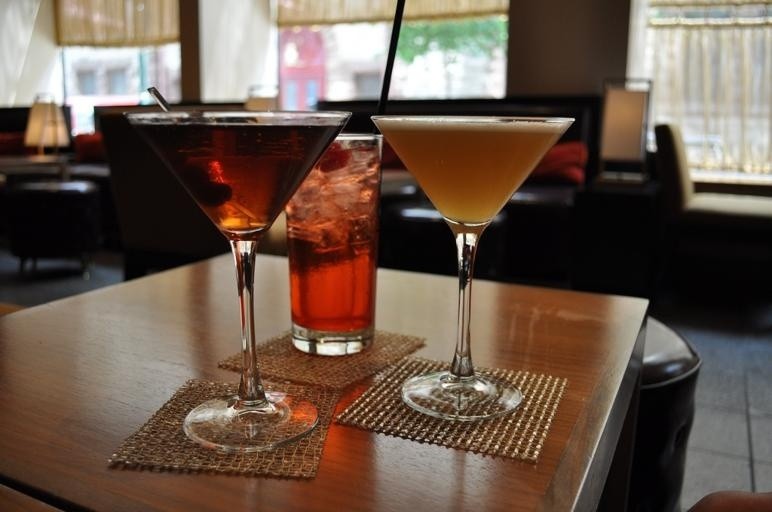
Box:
[73,131,105,160]
[0,131,37,155]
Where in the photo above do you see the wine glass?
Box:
[372,115,575,421]
[126,110,354,454]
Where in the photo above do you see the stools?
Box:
[601,314,705,511]
[9,179,99,276]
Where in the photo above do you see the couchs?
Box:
[97,106,258,284]
[312,95,604,284]
[655,124,772,322]
[70,150,115,187]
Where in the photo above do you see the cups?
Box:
[285,133,382,355]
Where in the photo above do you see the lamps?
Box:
[22,102,71,163]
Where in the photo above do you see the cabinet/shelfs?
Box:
[594,183,661,316]
